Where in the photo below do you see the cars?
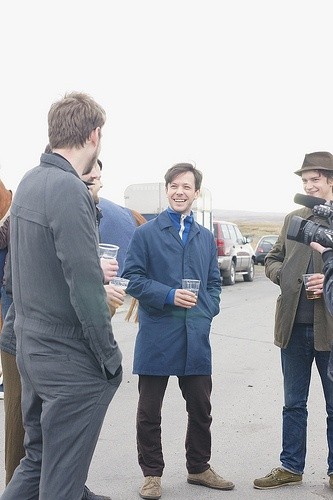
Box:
[254,234,279,266]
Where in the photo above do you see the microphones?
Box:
[293,193,333,210]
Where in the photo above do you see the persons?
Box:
[120,162,235,500]
[0,92,123,500]
[253,151,333,491]
[310,241,333,319]
[0,142,125,489]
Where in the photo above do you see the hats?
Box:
[294,151,333,177]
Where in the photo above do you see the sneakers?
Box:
[187,467,235,490]
[253,466,303,489]
[139,475,162,499]
[329,475,333,487]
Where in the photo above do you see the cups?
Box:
[109,277,130,308]
[302,273,322,299]
[98,243,120,259]
[181,279,200,305]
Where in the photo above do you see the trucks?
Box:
[123,180,215,238]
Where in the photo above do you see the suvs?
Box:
[212,220,257,286]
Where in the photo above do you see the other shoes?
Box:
[82,484,111,500]
